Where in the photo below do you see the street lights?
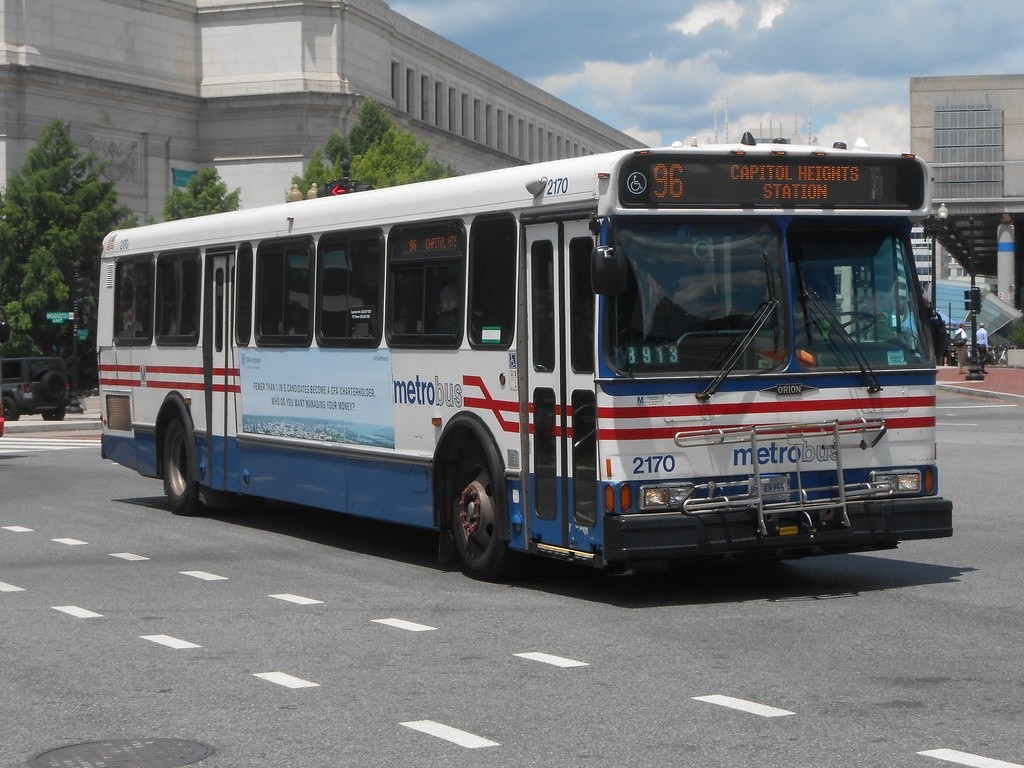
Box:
[921,203,948,314]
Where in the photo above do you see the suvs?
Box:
[0,357,70,422]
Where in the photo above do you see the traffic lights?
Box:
[316,179,374,197]
[965,289,982,310]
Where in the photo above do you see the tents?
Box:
[901,308,959,332]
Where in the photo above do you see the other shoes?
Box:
[981,369,988,374]
[958,371,967,374]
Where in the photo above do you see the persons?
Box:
[792,287,833,346]
[955,323,967,374]
[976,323,989,374]
[943,334,951,366]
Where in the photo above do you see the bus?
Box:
[96,132,953,584]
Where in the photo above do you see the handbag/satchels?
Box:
[953,330,966,347]
